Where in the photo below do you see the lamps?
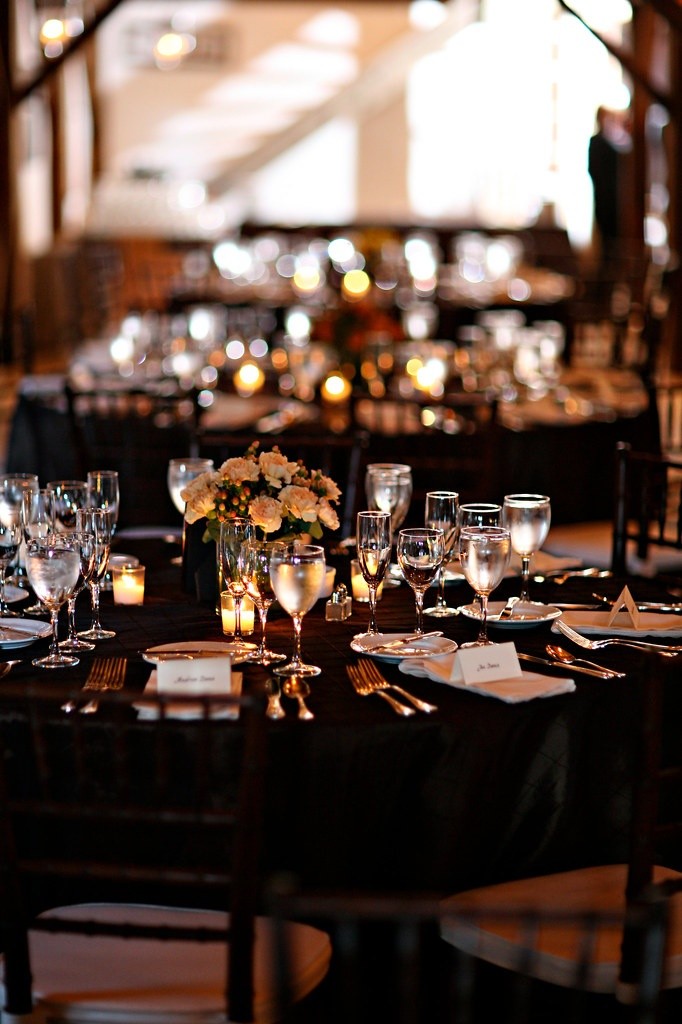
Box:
[350,558,383,600]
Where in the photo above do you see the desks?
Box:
[0,566,682,924]
[5,365,669,545]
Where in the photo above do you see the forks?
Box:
[555,620,682,658]
[510,566,614,584]
[60,659,127,714]
[347,657,439,718]
[592,592,682,614]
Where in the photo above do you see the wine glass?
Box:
[169,459,215,565]
[352,461,552,656]
[0,471,119,669]
[221,519,258,655]
[243,540,287,665]
[268,544,325,677]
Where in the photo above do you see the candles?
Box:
[114,566,145,606]
[220,591,255,636]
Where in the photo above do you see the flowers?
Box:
[183,440,343,593]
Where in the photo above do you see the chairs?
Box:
[0,235,682,1024]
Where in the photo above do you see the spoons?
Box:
[545,643,628,678]
[280,676,315,720]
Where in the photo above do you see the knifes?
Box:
[499,596,521,622]
[362,631,445,656]
[263,677,286,721]
[515,652,614,680]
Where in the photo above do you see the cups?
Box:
[220,591,256,636]
[351,559,383,602]
[112,564,145,606]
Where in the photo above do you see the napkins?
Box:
[397,652,576,704]
[551,611,682,638]
[132,667,242,721]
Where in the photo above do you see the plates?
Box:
[350,630,457,661]
[458,598,563,629]
[0,617,54,650]
